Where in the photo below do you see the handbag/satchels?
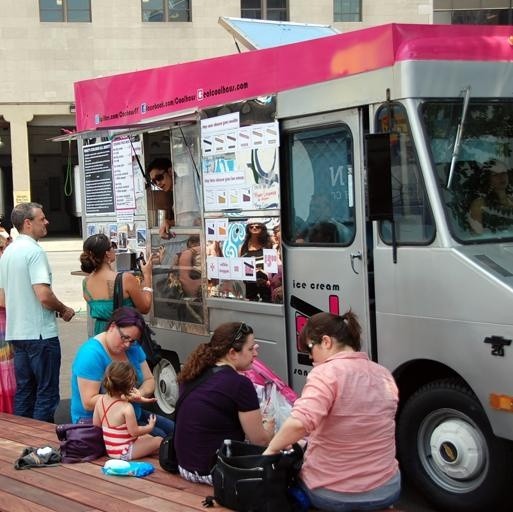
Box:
[210,441,303,511]
[159,436,179,474]
[55,422,105,464]
[140,336,181,381]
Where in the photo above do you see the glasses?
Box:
[118,328,135,345]
[149,174,164,183]
[250,225,261,229]
[107,241,118,252]
[302,341,317,353]
[229,321,250,349]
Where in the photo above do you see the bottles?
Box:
[221,439,233,458]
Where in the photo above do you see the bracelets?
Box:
[141,285,155,294]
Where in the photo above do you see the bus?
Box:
[44,17,513,509]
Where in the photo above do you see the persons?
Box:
[170,320,278,487]
[259,308,403,511]
[0,201,76,424]
[469,158,513,230]
[67,305,177,443]
[91,359,165,462]
[144,156,175,241]
[306,193,351,244]
[0,206,21,415]
[154,222,280,318]
[78,232,156,339]
